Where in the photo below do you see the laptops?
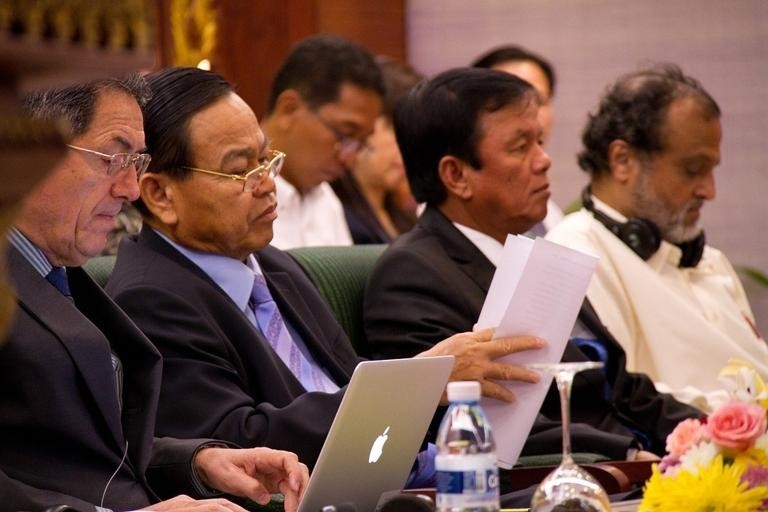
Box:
[296,355,455,511]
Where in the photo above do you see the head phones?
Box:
[582,188,705,269]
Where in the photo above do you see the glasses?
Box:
[171,148,285,190]
[303,107,375,160]
[66,144,152,178]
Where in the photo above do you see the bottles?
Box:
[435,382,501,511]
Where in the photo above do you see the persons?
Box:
[320,56,427,243]
[417,47,566,240]
[537,67,766,459]
[363,70,705,462]
[104,68,544,474]
[251,37,386,249]
[2,64,310,511]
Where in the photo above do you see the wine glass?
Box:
[526,363,614,512]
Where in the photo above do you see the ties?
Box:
[48,266,124,423]
[251,275,470,490]
